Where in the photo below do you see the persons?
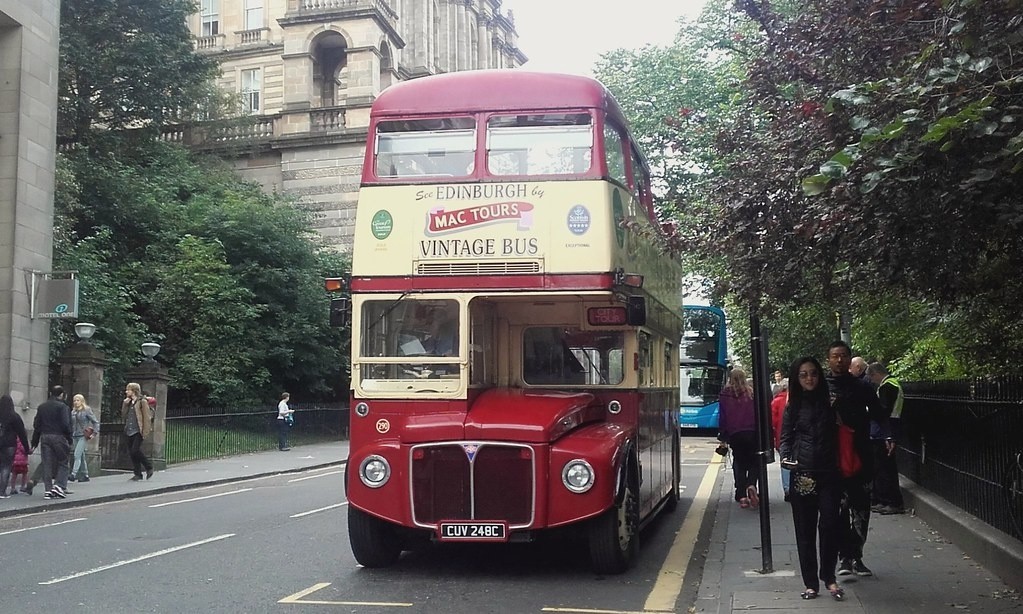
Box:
[770,369,790,453]
[825,340,898,575]
[68,394,100,482]
[277,392,295,451]
[419,308,460,379]
[865,362,905,514]
[0,385,74,499]
[121,383,154,481]
[848,356,871,384]
[780,357,845,601]
[718,368,759,510]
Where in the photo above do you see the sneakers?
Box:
[43,491,56,499]
[50,485,66,498]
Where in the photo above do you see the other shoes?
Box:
[837,561,851,574]
[746,485,759,508]
[800,589,817,600]
[279,448,289,451]
[870,503,884,511]
[18,488,27,495]
[78,475,89,482]
[880,506,906,515]
[9,489,18,495]
[67,475,74,483]
[825,582,843,600]
[739,498,749,509]
[0,495,10,499]
[146,466,153,480]
[131,475,143,481]
[26,481,32,496]
[852,561,872,576]
[64,487,74,494]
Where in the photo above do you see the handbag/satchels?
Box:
[837,421,861,474]
[83,427,95,440]
[788,468,823,499]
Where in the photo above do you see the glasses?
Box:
[798,369,819,379]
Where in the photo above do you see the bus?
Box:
[680,305,733,437]
[326,68,683,578]
